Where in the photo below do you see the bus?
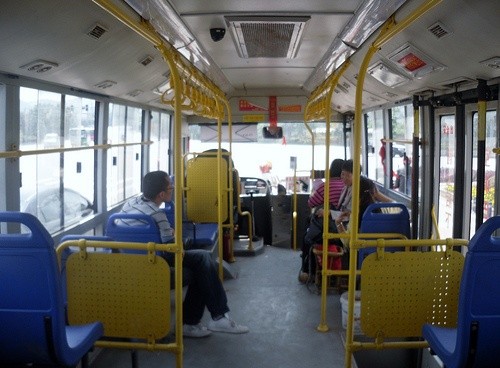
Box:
[70,127,94,146]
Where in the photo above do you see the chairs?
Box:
[422,213,500,368]
[358,203,411,280]
[0,149,238,368]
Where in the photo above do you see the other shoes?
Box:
[297,271,309,282]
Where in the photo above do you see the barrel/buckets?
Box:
[340,290,362,330]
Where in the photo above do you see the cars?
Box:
[42,133,60,148]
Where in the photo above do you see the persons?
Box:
[114,170,249,338]
[299,159,382,284]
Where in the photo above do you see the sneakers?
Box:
[209,313,249,334]
[172,322,209,339]
